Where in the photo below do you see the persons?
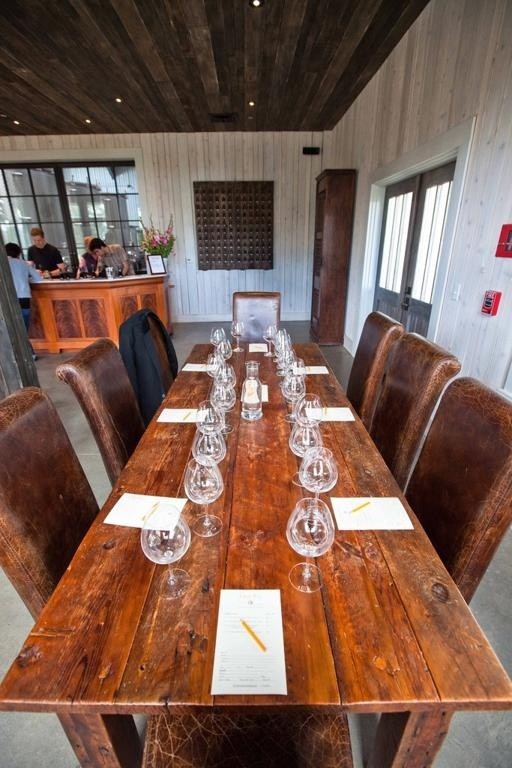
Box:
[88,237,135,275]
[4,242,42,361]
[77,235,107,278]
[25,228,66,278]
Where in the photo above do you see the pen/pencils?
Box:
[349,502,371,514]
[325,405,327,417]
[238,617,267,652]
[142,502,159,520]
[183,412,191,420]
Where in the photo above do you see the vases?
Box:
[138,215,176,258]
[163,259,168,273]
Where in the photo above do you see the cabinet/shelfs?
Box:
[309,170,356,346]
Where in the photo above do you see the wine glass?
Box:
[285,496,337,596]
[182,319,339,522]
[181,455,223,539]
[142,505,195,600]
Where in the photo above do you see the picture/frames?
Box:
[147,255,166,275]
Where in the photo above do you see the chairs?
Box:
[120,309,176,427]
[233,293,281,344]
[56,339,146,488]
[369,333,461,493]
[405,376,511,605]
[347,311,404,433]
[0,386,100,623]
